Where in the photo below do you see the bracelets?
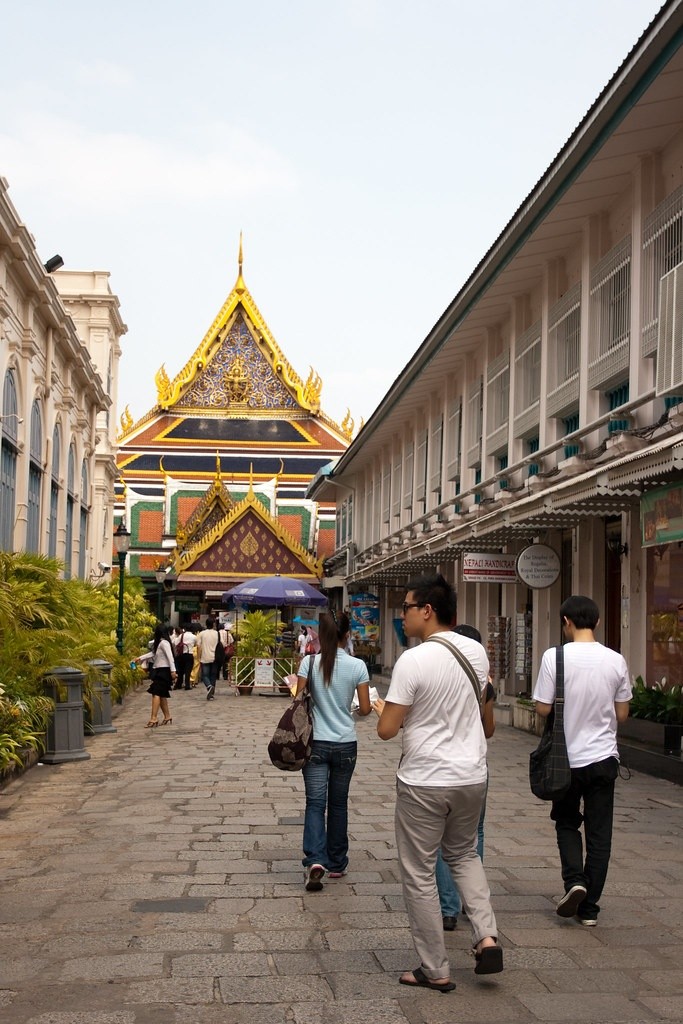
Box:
[138,658,141,661]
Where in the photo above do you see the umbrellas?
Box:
[290,616,319,625]
[307,627,321,655]
[221,574,327,659]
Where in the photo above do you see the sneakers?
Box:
[328,866,348,877]
[556,884,587,918]
[305,864,325,891]
[578,908,597,925]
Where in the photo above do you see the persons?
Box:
[295,624,355,660]
[433,623,496,931]
[368,574,503,991]
[534,597,633,927]
[294,610,374,890]
[132,618,234,729]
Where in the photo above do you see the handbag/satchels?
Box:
[529,724,572,801]
[176,633,189,657]
[213,642,228,668]
[225,645,234,659]
[268,655,315,771]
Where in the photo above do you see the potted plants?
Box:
[511,697,535,734]
[611,673,683,750]
[227,645,301,697]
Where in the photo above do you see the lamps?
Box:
[44,255,64,273]
[607,531,628,562]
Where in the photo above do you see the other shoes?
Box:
[187,688,192,690]
[443,916,456,931]
[206,686,214,701]
[192,685,196,688]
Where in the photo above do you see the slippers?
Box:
[474,946,504,975]
[399,967,456,991]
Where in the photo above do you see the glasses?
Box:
[402,601,436,615]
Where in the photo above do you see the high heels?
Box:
[160,718,172,725]
[144,721,159,728]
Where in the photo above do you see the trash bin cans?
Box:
[34,666,92,765]
[78,658,118,735]
